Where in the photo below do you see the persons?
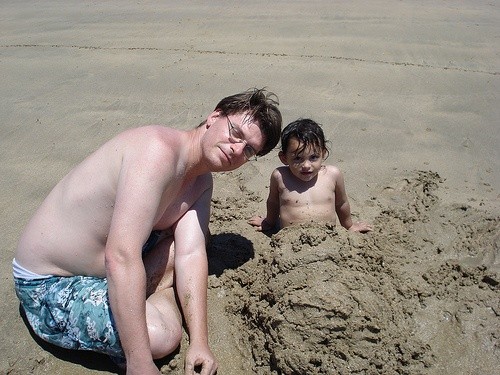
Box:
[14,87,283,374]
[248,117,372,235]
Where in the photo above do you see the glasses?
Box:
[226,114,258,162]
